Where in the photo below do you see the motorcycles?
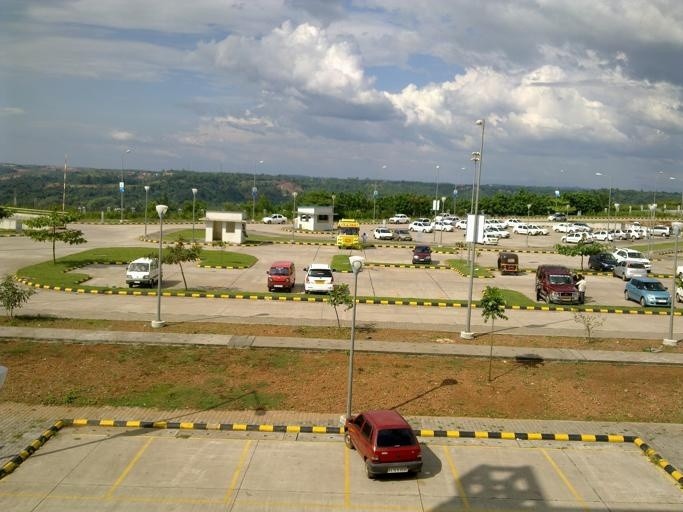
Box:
[497,251,520,276]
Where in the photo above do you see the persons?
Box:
[573,274,585,304]
[361,232,368,244]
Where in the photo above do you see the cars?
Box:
[623,276,672,308]
[676,287,683,303]
[412,246,433,265]
[588,252,619,272]
[669,175,681,181]
[676,265,683,276]
[344,408,423,479]
[262,213,287,224]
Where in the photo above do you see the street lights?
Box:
[144,186,149,239]
[120,149,131,221]
[467,151,480,267]
[467,118,486,333]
[440,196,447,245]
[252,160,264,222]
[613,203,620,242]
[652,203,657,252]
[654,170,664,204]
[292,192,298,241]
[565,205,570,248]
[155,205,168,321]
[373,165,387,223]
[647,206,655,257]
[346,255,366,420]
[526,204,532,248]
[556,169,564,212]
[596,173,612,242]
[669,221,683,339]
[332,194,336,237]
[192,189,197,242]
[434,166,440,241]
[453,167,465,215]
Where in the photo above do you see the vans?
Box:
[612,260,648,281]
[126,257,159,288]
[267,258,295,293]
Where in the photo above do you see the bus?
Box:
[337,217,361,250]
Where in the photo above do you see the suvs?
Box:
[535,264,579,306]
[303,263,337,294]
[611,248,653,273]
